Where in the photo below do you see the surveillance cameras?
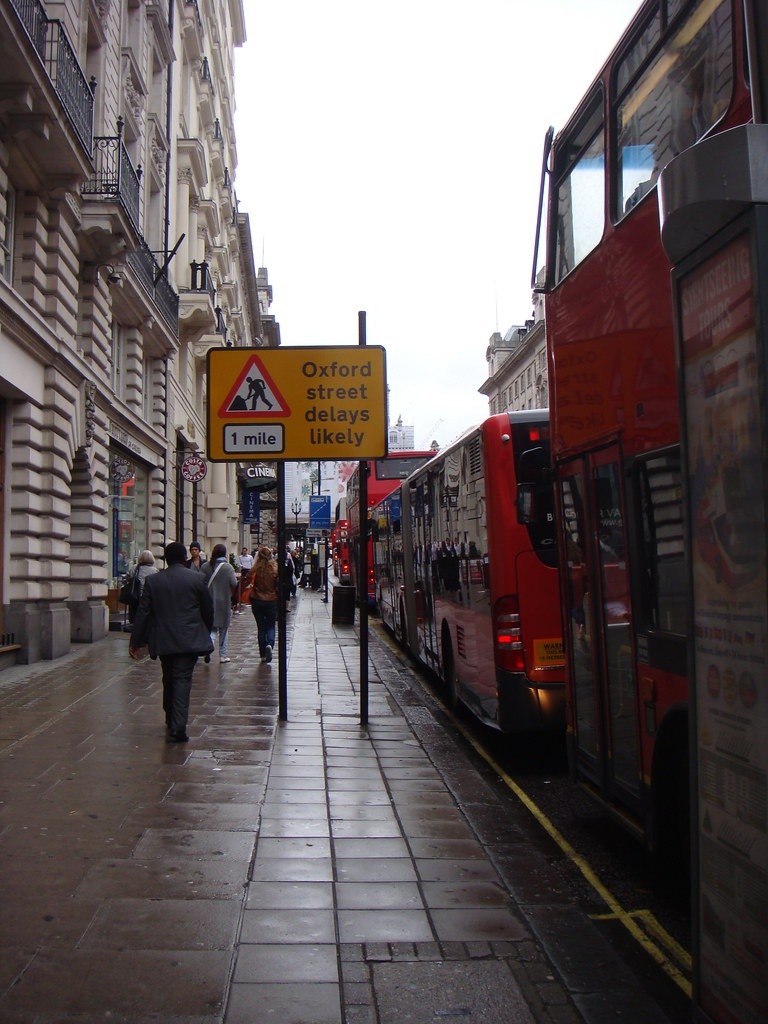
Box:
[108,273,121,283]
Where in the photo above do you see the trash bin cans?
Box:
[332,586,355,625]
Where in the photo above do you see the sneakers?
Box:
[220,656,230,662]
[204,654,211,663]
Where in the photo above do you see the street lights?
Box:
[291,497,302,550]
[312,477,333,496]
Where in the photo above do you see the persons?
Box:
[125,540,313,663]
[129,542,214,747]
[391,538,479,602]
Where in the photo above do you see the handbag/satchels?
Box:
[119,564,141,604]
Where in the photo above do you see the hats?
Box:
[190,541,201,552]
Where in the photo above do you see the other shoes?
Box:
[261,658,266,661]
[298,582,302,585]
[166,735,190,742]
[299,585,305,588]
[286,600,291,611]
[266,645,273,663]
[129,643,139,660]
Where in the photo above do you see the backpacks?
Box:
[254,565,276,594]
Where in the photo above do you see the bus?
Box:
[285,519,347,550]
[367,407,565,746]
[346,451,437,601]
[331,497,350,584]
[534,0,768,879]
